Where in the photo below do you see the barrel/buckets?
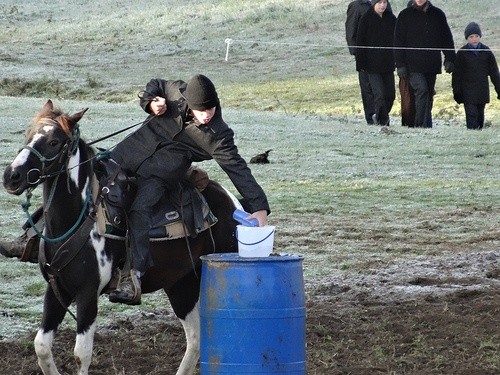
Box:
[234,225,276,257]
[199,253,306,375]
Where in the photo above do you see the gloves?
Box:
[444,61,455,73]
[396,67,408,78]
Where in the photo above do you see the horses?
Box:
[0,98,249,375]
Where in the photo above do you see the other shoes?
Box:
[109,276,141,305]
[0,235,40,263]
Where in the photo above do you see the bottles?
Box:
[232,209,259,227]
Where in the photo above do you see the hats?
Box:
[186,74,218,110]
[464,22,482,39]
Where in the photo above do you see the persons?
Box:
[344,0,456,129]
[444,21,500,131]
[82,72,274,306]
